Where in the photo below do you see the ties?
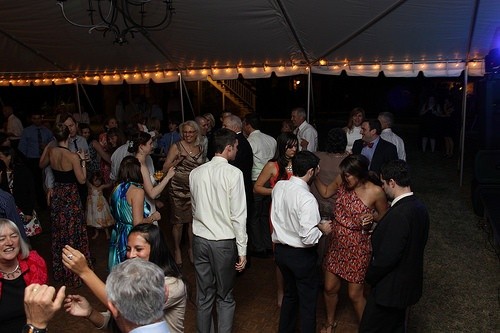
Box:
[73,139,79,151]
[296,128,299,135]
[37,128,43,155]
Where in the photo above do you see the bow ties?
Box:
[363,142,374,148]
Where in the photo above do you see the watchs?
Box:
[21,323,49,333]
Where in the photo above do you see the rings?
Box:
[370,220,372,224]
[67,253,73,259]
[32,288,38,291]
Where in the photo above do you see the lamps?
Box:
[56,0,176,46]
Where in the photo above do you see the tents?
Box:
[0,0,499,188]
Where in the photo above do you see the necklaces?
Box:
[286,160,293,171]
[189,150,193,153]
[1,264,21,279]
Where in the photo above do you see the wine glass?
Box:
[154,170,164,185]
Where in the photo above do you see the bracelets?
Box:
[85,309,93,319]
[81,165,86,167]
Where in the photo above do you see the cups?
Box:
[321,216,330,236]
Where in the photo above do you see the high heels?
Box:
[320,321,337,333]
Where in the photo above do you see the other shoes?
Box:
[190,260,194,265]
[177,263,182,268]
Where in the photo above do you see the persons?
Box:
[0,103,431,332]
